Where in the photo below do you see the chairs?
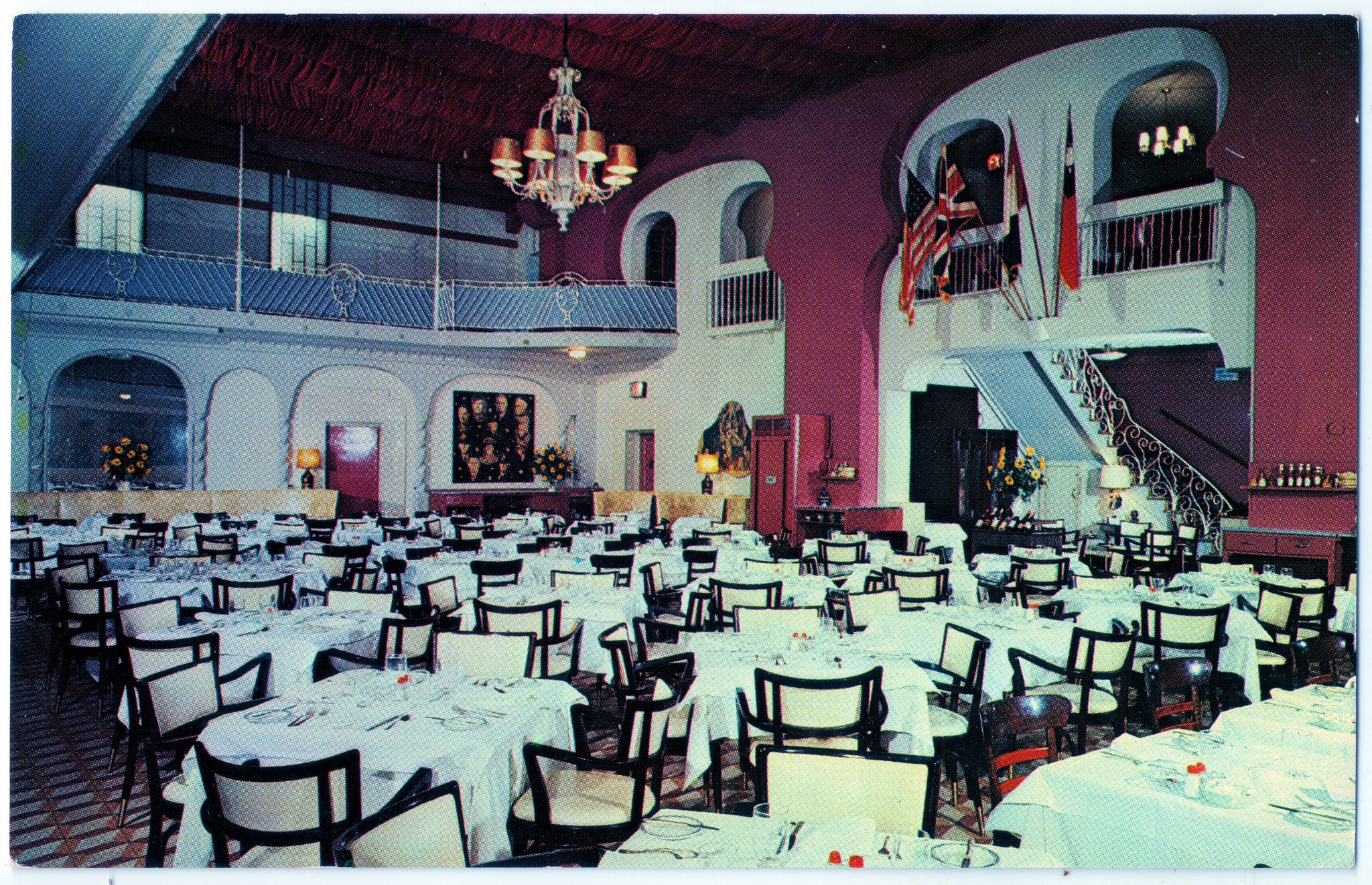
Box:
[11,511,1358,868]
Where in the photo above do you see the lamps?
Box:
[295,448,322,489]
[1138,88,1197,157]
[697,452,721,495]
[491,13,637,233]
[1098,462,1132,510]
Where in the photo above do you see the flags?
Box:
[896,114,1081,327]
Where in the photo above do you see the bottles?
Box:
[790,632,808,652]
[1257,463,1332,488]
[998,511,1040,532]
[1183,763,1208,799]
[975,508,1004,530]
[1027,605,1039,622]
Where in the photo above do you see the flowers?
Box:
[533,440,574,486]
[102,437,152,477]
[985,447,1048,510]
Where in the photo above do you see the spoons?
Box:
[834,657,842,668]
[288,707,330,726]
[385,714,411,730]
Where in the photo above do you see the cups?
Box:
[384,654,459,698]
[229,594,324,624]
[891,824,930,868]
[1280,727,1314,779]
[1198,730,1229,762]
[752,803,790,868]
[1263,565,1294,585]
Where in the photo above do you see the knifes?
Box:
[961,837,974,868]
[366,713,400,731]
[647,817,721,831]
[1267,804,1354,823]
[775,822,805,853]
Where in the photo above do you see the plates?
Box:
[931,843,1000,868]
[1290,804,1355,830]
[244,709,292,723]
[739,655,771,664]
[441,716,487,732]
[641,815,704,841]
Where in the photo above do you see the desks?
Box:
[1054,584,1273,704]
[87,559,327,682]
[866,603,1093,699]
[460,584,646,684]
[597,809,1063,867]
[1209,677,1357,753]
[10,523,112,573]
[1221,526,1345,585]
[1170,567,1357,640]
[979,728,1356,867]
[169,667,586,869]
[115,606,405,731]
[682,621,943,812]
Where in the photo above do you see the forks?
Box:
[619,849,699,860]
